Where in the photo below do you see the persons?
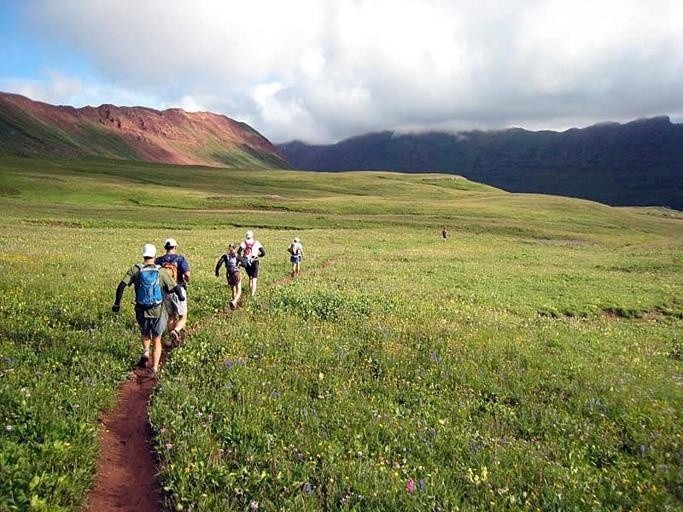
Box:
[110,241,184,377]
[440,228,448,242]
[213,243,249,311]
[236,229,264,297]
[153,237,190,349]
[285,237,304,277]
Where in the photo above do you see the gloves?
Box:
[112,304,120,313]
[179,295,185,301]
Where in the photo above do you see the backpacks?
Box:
[133,263,163,307]
[243,240,256,262]
[227,257,236,274]
[161,255,180,282]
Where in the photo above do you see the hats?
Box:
[246,230,253,238]
[142,244,156,258]
[164,239,177,247]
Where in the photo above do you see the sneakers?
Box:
[169,330,181,347]
[137,350,149,369]
[230,301,237,311]
